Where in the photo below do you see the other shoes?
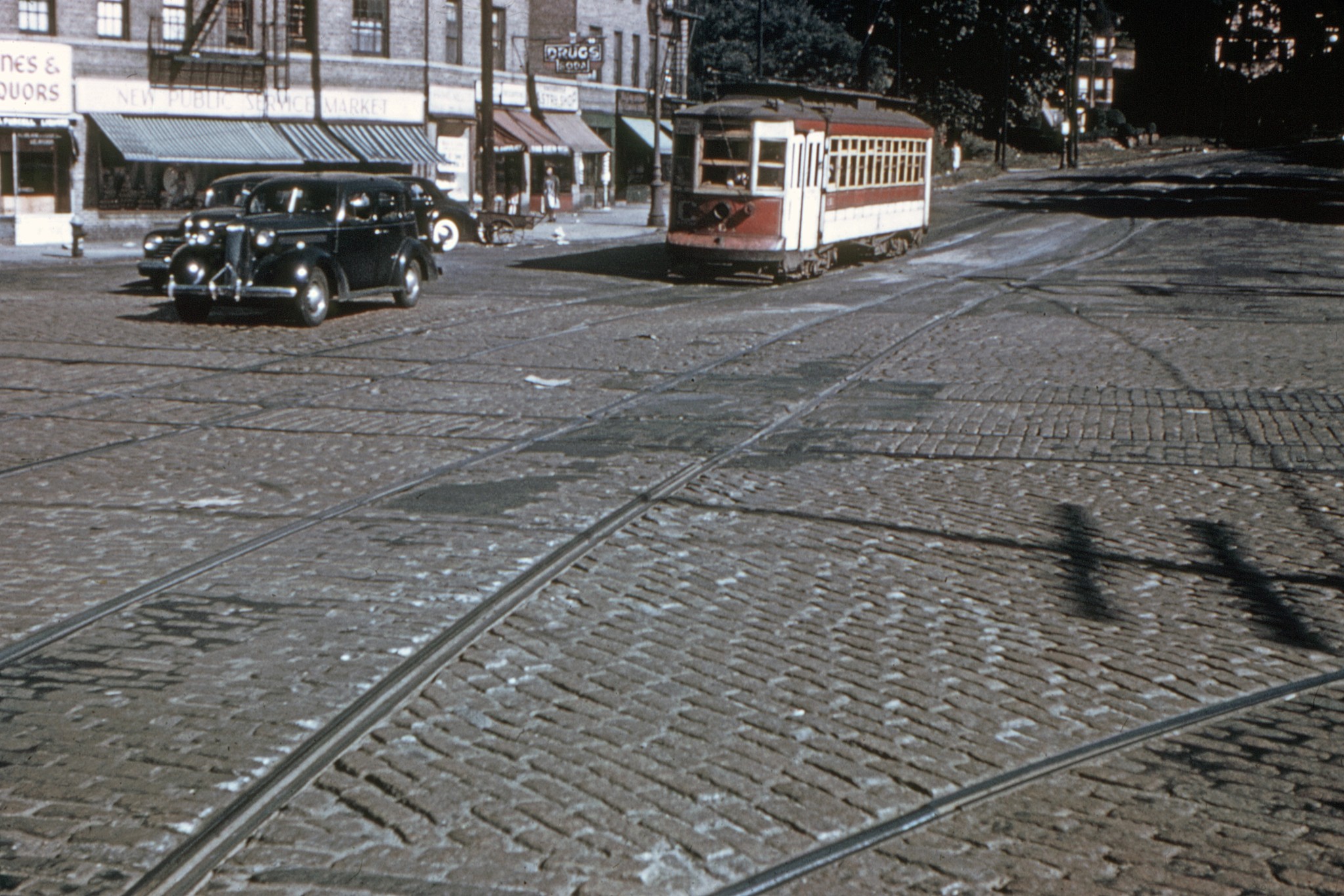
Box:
[548,217,557,224]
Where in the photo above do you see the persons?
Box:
[542,167,560,222]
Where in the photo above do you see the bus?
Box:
[668,64,933,286]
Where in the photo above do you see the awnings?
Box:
[271,118,456,167]
[477,112,526,154]
[89,112,304,167]
[621,115,672,157]
[494,108,568,155]
[540,109,613,154]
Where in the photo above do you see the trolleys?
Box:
[475,205,549,246]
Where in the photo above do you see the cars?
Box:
[138,169,478,328]
